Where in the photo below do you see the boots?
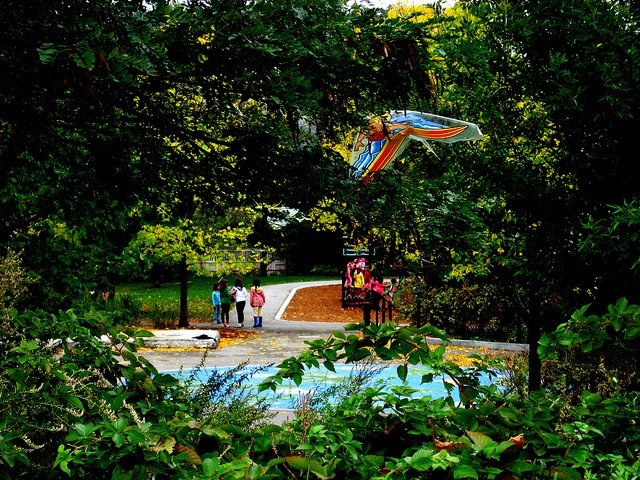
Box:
[258,317,262,327]
[252,316,258,327]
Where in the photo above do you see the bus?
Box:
[190,247,287,277]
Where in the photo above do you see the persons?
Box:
[250,279,265,327]
[212,283,221,324]
[231,278,248,328]
[220,279,237,327]
[338,265,398,310]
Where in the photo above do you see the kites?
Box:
[349,111,484,191]
